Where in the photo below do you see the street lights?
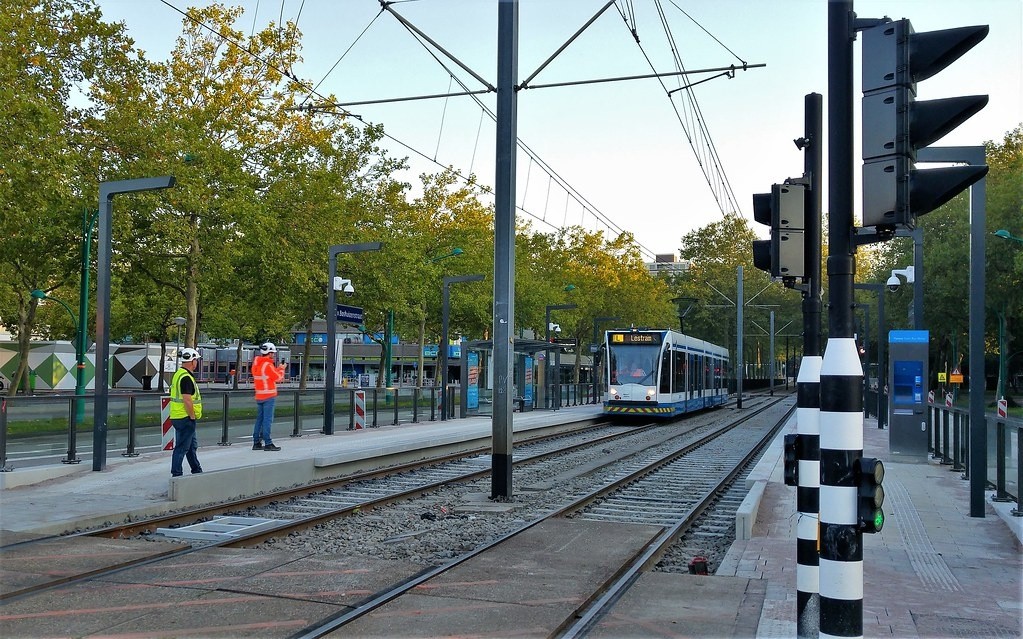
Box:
[518,284,577,339]
[440,275,486,421]
[174,314,186,373]
[854,283,885,430]
[544,304,578,409]
[853,304,870,419]
[384,249,464,404]
[92,175,178,472]
[321,345,328,386]
[592,317,621,404]
[298,353,303,381]
[322,242,384,432]
[31,153,201,426]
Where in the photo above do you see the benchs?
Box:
[480,395,531,405]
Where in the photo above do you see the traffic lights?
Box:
[854,454,886,533]
[752,182,805,277]
[863,17,990,228]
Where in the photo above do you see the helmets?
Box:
[260,342,277,355]
[181,348,202,363]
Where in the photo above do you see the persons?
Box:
[169,348,203,477]
[629,363,647,376]
[251,343,287,451]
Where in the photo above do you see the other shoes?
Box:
[264,444,281,451]
[252,443,265,450]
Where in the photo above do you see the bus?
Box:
[595,296,730,424]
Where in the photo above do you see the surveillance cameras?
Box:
[887,277,901,292]
[343,285,354,298]
[555,327,561,335]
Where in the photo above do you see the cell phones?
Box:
[282,358,285,364]
